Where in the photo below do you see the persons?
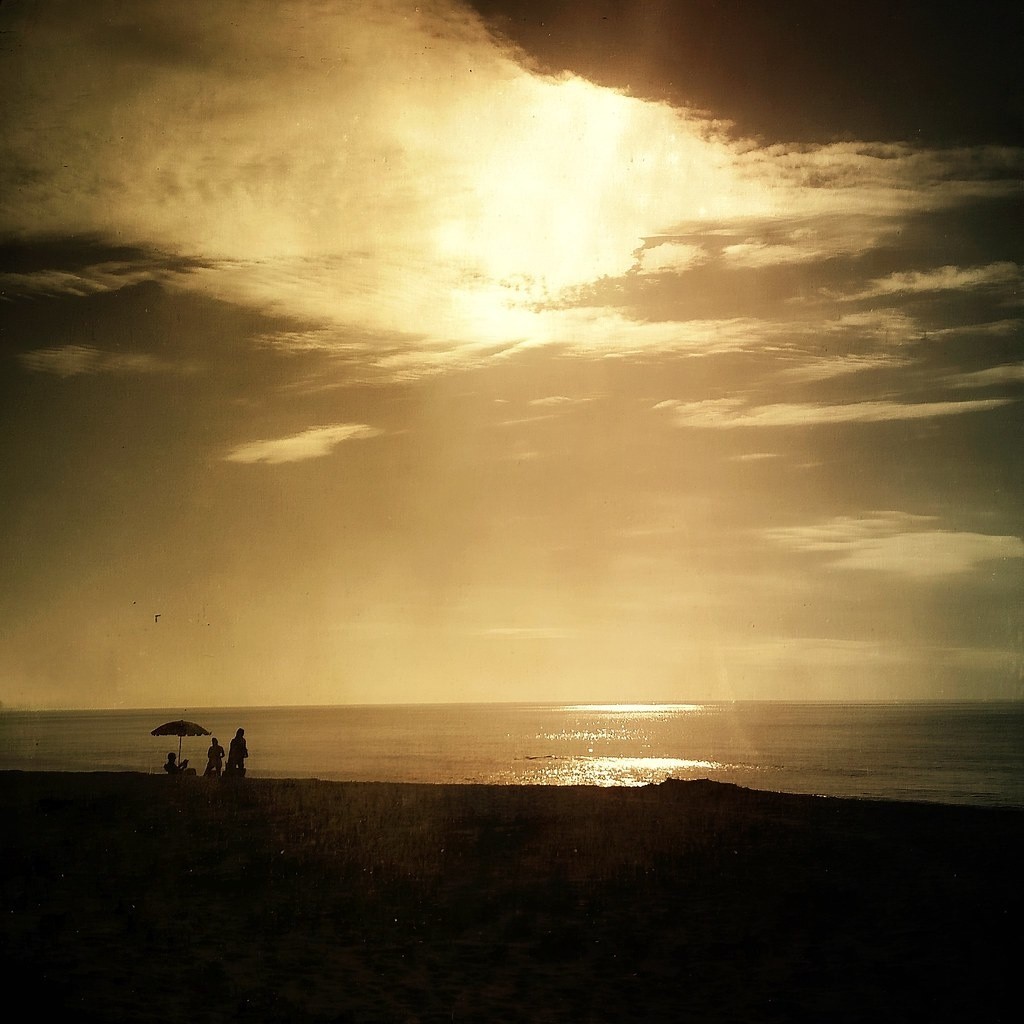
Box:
[202,736,224,775]
[228,728,250,770]
[165,752,197,775]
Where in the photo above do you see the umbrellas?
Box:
[150,720,212,765]
